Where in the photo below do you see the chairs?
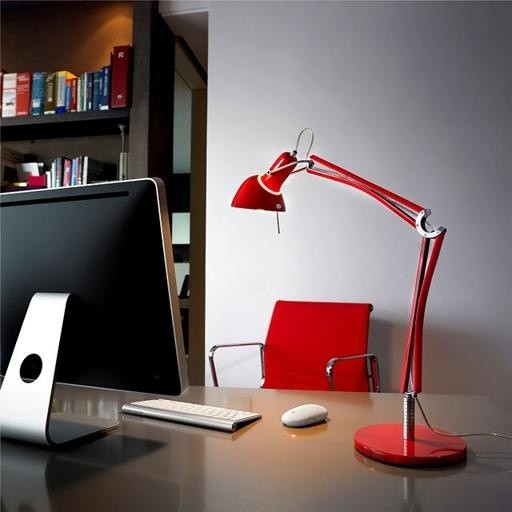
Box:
[206,299,385,393]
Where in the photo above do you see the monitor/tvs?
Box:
[1,176,188,447]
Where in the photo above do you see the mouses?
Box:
[281,404,329,427]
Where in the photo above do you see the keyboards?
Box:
[122,398,264,432]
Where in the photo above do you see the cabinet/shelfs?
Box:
[0,1,175,233]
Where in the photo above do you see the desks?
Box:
[0,384,511,512]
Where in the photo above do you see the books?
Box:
[31,71,45,116]
[17,74,31,116]
[111,45,130,109]
[57,66,108,112]
[44,71,57,113]
[2,74,17,118]
[45,157,101,188]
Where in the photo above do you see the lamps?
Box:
[230,128,469,468]
